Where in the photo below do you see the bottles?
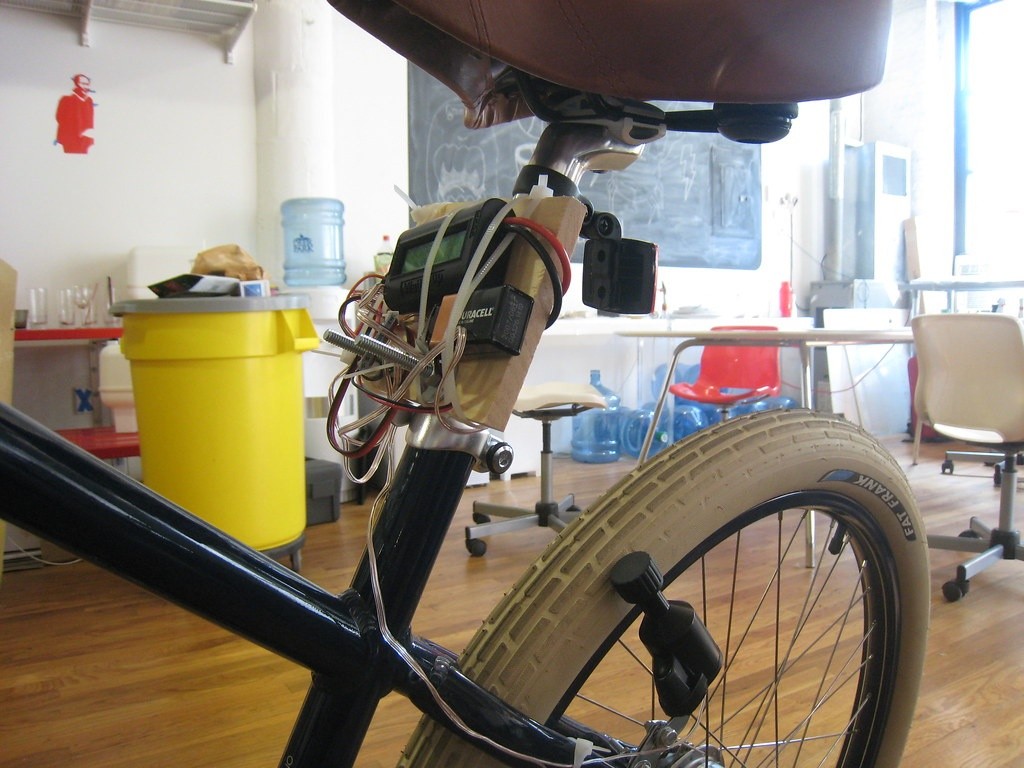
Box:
[619,406,669,459]
[641,362,800,444]
[570,369,622,463]
[280,198,348,288]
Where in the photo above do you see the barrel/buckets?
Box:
[108,293,321,552]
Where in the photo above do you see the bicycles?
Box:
[0,0,929,768]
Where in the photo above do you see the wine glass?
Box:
[71,283,93,328]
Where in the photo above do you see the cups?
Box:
[27,288,48,329]
[101,287,122,327]
[55,289,77,328]
[14,309,28,328]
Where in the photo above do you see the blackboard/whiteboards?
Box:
[406,55,765,271]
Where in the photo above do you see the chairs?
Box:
[909,312,1024,602]
[667,326,782,424]
[464,380,610,558]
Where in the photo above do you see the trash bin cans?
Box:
[109,291,321,575]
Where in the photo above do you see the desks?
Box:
[900,277,1024,468]
[13,327,127,466]
[614,326,913,569]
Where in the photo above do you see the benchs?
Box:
[48,425,143,460]
